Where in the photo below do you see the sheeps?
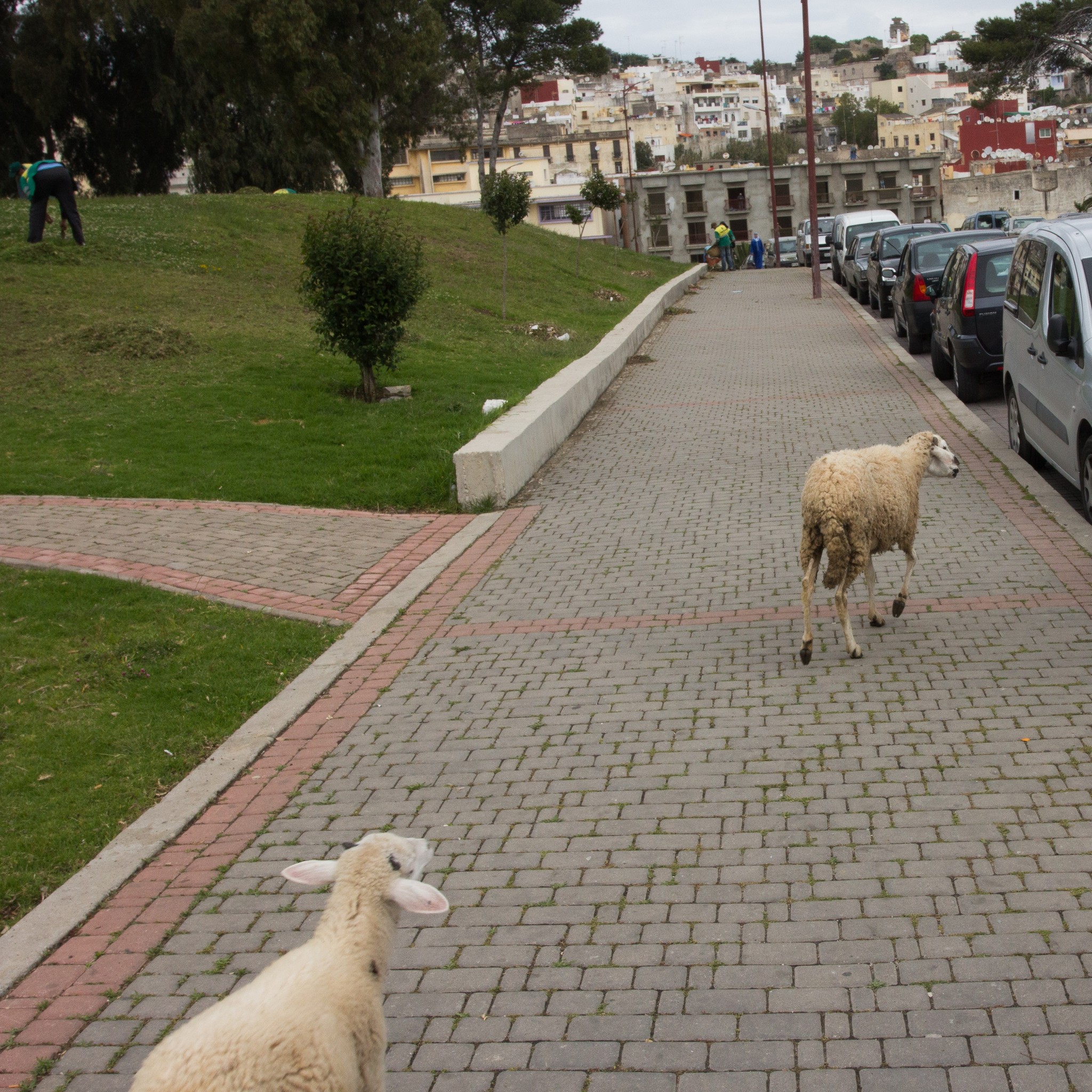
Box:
[798,430,959,665]
[129,830,449,1092]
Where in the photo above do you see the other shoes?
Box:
[719,269,725,271]
[726,268,733,271]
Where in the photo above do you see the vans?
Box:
[766,236,799,267]
[795,216,836,269]
[1001,212,1092,525]
[960,211,1011,233]
[1001,216,1046,235]
[830,210,901,285]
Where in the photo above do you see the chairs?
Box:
[922,253,940,268]
[886,238,900,251]
[986,261,997,279]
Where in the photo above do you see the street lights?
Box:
[741,102,780,268]
[621,77,651,252]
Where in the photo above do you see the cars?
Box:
[739,254,765,269]
[842,222,1020,404]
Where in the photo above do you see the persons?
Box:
[923,215,931,223]
[7,159,86,247]
[750,232,765,269]
[737,193,743,210]
[777,223,782,237]
[710,221,736,272]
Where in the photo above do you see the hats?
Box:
[711,222,717,228]
[8,162,21,178]
